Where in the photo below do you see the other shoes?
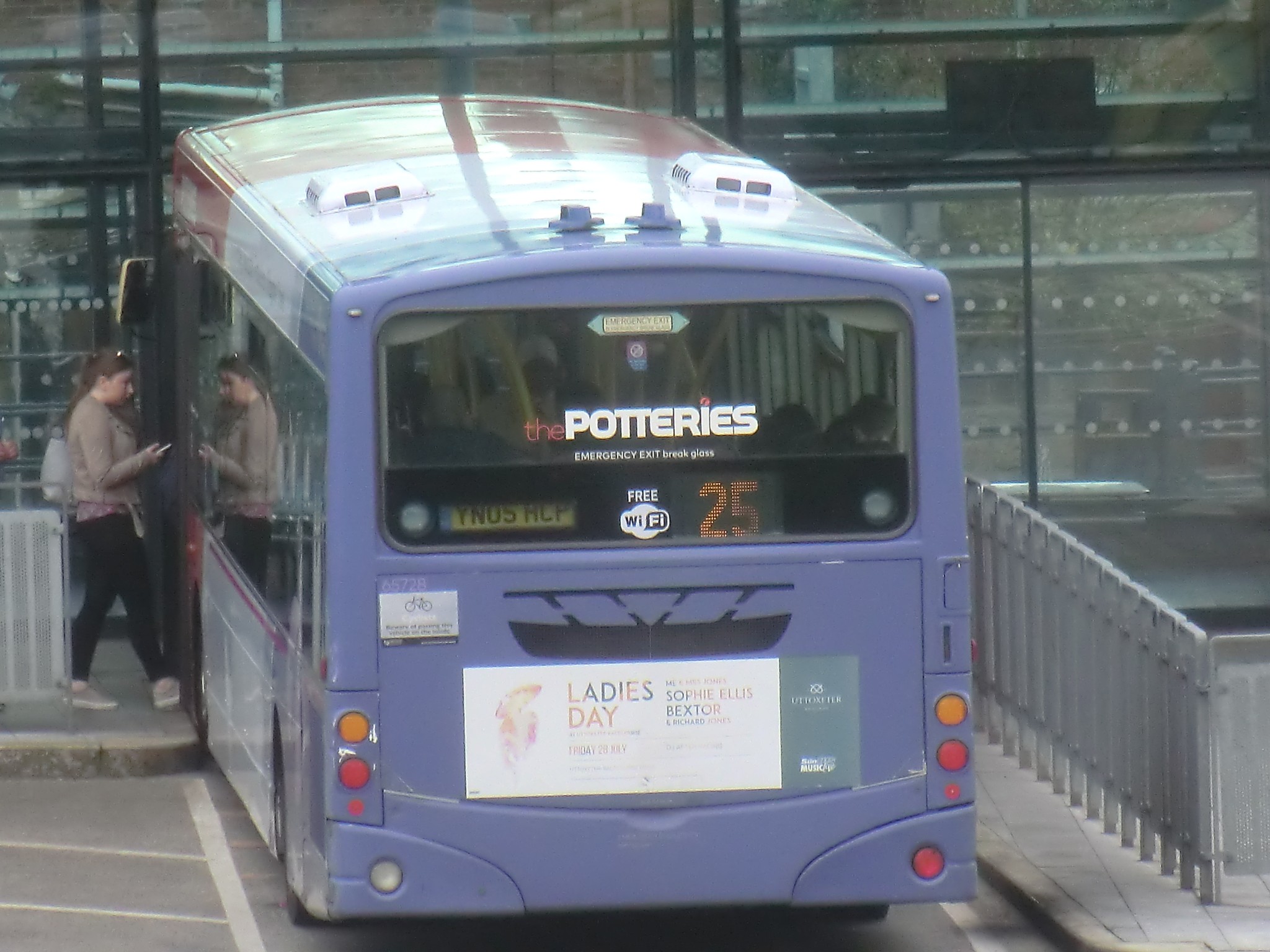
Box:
[155,676,181,708]
[63,685,117,710]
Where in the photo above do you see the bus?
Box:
[113,93,983,928]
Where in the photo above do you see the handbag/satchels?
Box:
[129,503,148,538]
[204,510,225,541]
[40,438,73,505]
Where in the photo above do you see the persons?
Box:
[752,395,907,536]
[64,349,183,709]
[196,353,278,590]
[389,349,616,468]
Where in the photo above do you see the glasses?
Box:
[102,350,124,377]
[230,350,257,374]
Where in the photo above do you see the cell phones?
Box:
[154,442,172,454]
